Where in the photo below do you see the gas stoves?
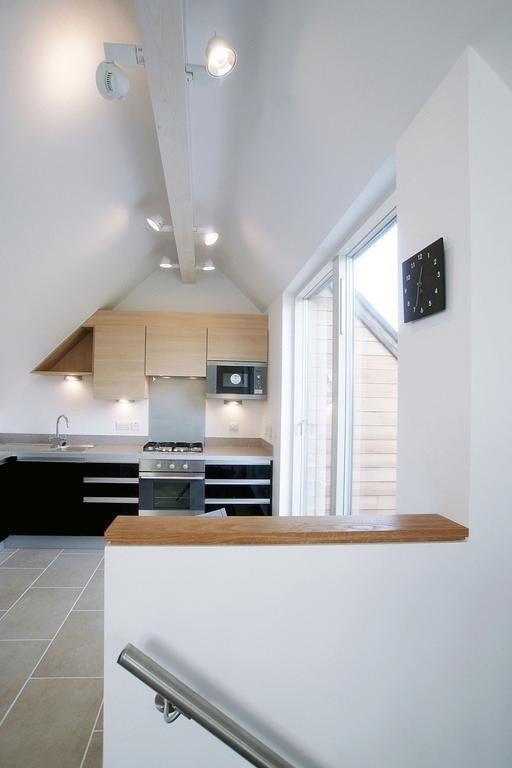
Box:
[140,440,204,455]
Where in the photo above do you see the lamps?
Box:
[147,212,180,269]
[97,32,238,102]
[195,223,220,272]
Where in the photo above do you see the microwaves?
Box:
[206,361,267,400]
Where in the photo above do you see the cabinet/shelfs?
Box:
[0,462,273,542]
[30,310,271,401]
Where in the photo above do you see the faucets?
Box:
[55,415,69,438]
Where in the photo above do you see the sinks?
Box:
[41,444,96,455]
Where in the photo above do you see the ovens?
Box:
[139,459,205,517]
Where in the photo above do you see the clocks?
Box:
[397,238,453,324]
